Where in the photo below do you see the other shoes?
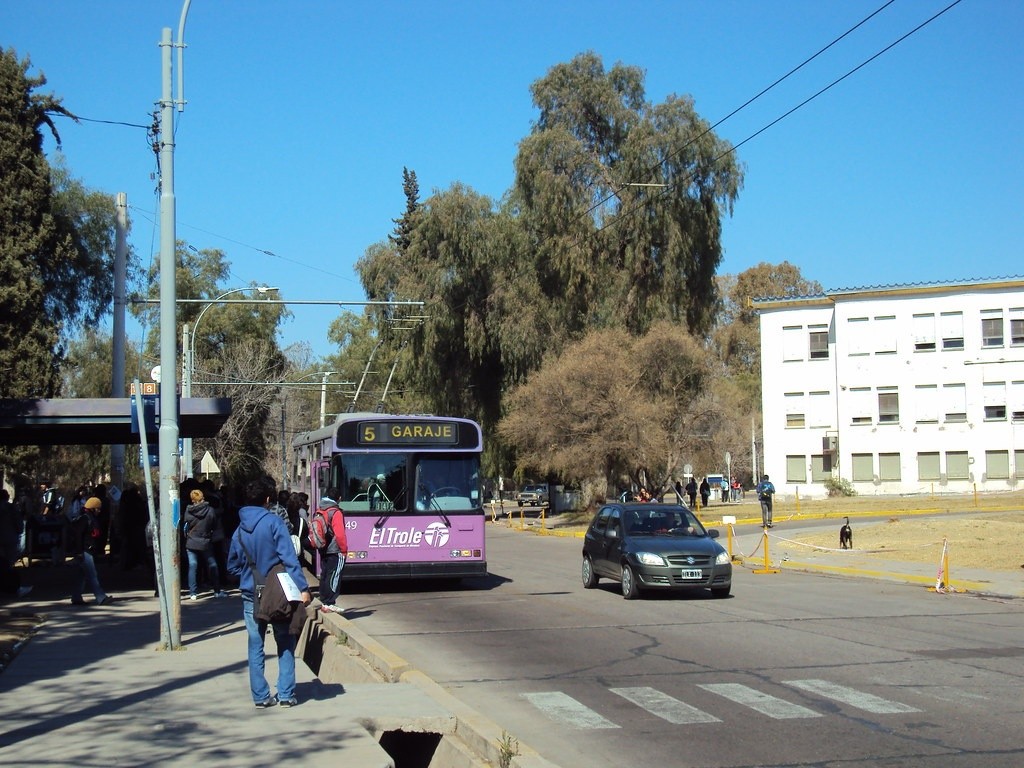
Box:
[191,595,197,600]
[322,603,344,612]
[101,596,112,605]
[767,523,773,528]
[72,601,90,606]
[214,591,229,598]
[279,698,298,707]
[256,697,277,709]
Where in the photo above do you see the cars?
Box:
[582,503,733,600]
[517,484,548,507]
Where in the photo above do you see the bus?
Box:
[291,412,496,590]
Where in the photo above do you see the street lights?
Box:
[281,371,338,491]
[182,286,280,480]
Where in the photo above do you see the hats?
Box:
[84,497,101,509]
[190,489,203,502]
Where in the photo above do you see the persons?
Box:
[267,486,315,560]
[756,475,776,528]
[659,512,697,534]
[676,481,682,505]
[40,479,57,552]
[320,487,348,613]
[721,478,729,502]
[65,478,228,605]
[731,476,740,503]
[228,481,310,708]
[0,489,24,601]
[634,487,659,503]
[700,478,710,507]
[536,487,543,492]
[685,478,697,507]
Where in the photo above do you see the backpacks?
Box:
[759,482,770,501]
[307,508,338,548]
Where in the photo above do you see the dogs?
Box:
[840,516,853,549]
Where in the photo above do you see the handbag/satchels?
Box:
[290,534,300,556]
[253,584,266,623]
[705,490,711,496]
